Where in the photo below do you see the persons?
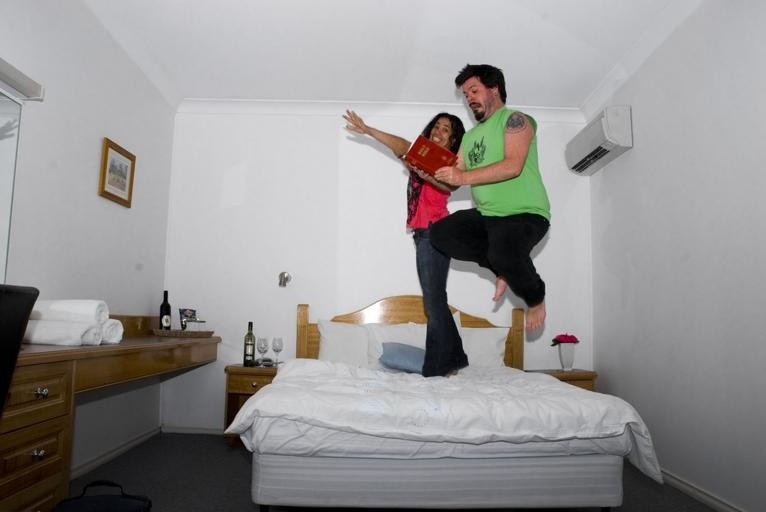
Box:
[342,108,470,377]
[407,61,552,332]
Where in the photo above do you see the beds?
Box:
[249,295,624,511]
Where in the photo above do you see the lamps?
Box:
[278,272,292,287]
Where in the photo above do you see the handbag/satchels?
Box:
[52,480,153,512]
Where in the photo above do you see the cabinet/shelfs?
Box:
[0,334,221,512]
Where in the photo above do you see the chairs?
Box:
[1,284,40,419]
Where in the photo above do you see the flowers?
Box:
[549,332,579,346]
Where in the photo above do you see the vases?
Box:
[558,343,575,370]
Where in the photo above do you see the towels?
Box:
[28,299,108,323]
[23,319,103,345]
[102,319,124,344]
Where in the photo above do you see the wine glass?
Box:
[257,334,283,368]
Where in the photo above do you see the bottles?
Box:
[242,321,256,368]
[158,291,172,331]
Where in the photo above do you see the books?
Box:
[397,134,459,178]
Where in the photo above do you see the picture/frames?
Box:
[98,136,136,208]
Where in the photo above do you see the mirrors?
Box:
[0,89,23,284]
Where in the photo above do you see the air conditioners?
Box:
[565,105,632,177]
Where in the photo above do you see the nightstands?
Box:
[525,369,597,391]
[224,365,278,432]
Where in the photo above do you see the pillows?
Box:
[315,311,510,376]
